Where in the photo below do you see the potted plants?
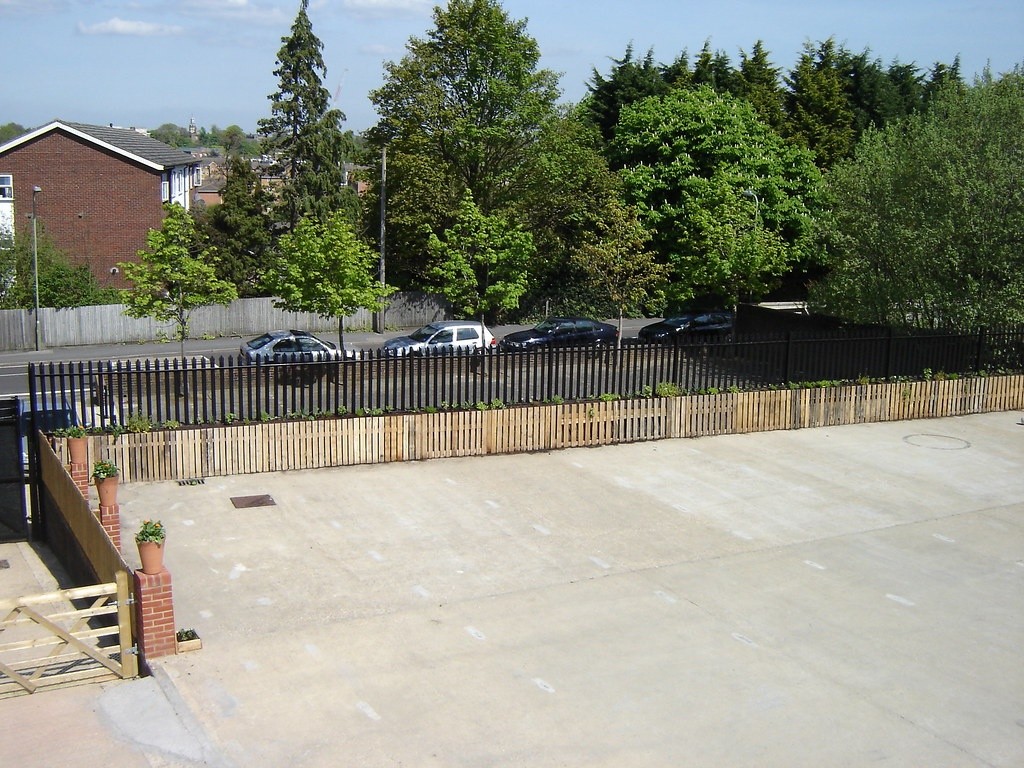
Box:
[175,629,203,654]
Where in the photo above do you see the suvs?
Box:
[382,319,499,360]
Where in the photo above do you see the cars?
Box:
[500,317,620,352]
[238,329,366,367]
[636,311,737,343]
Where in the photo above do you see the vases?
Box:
[67,438,87,463]
[94,474,120,506]
[136,535,166,574]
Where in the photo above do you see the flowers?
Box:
[65,425,89,438]
[89,458,121,483]
[134,517,166,546]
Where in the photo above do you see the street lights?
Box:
[740,190,758,240]
[33,186,43,348]
